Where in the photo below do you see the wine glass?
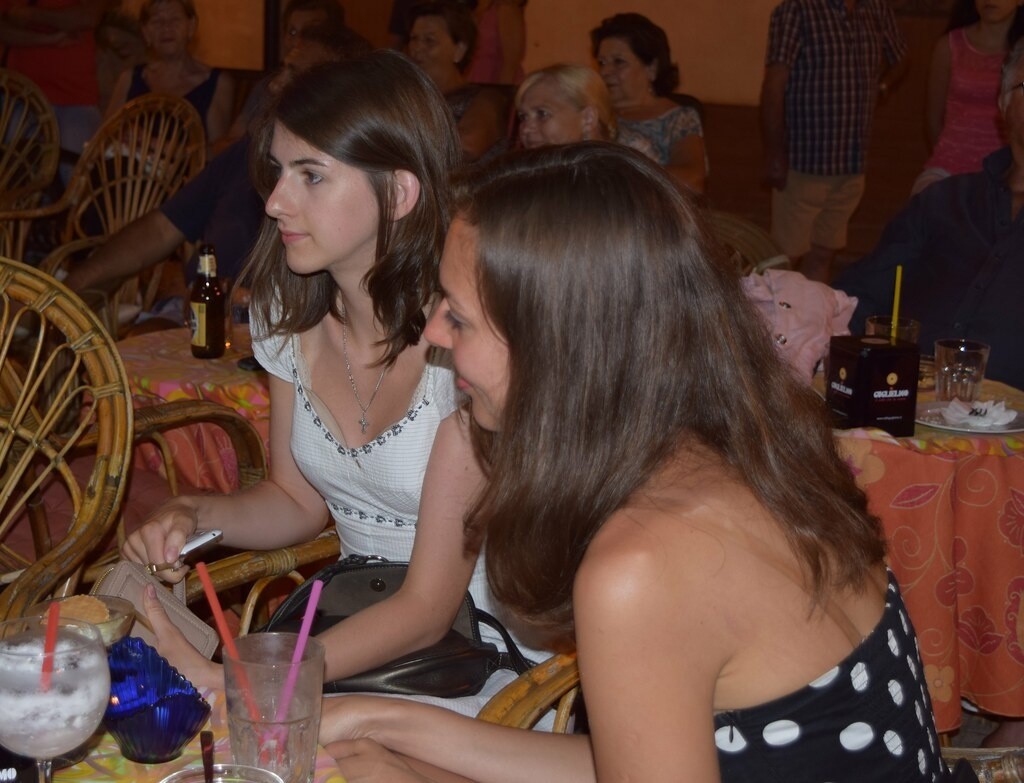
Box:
[0,617,110,783]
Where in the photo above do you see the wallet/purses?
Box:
[88,560,221,661]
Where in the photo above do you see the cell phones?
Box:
[179,530,226,559]
[237,356,264,370]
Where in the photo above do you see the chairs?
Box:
[0,31,789,734]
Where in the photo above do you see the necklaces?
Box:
[337,302,391,435]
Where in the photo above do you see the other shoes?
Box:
[5,323,29,342]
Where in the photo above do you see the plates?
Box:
[914,401,1024,433]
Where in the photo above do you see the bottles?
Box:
[190,244,226,358]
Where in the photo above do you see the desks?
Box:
[807,357,1024,744]
[76,325,272,496]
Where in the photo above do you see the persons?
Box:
[515,62,610,150]
[212,0,343,158]
[315,142,954,783]
[0,0,233,234]
[388,0,529,159]
[120,49,486,689]
[31,23,379,341]
[911,0,1024,199]
[589,13,709,194]
[830,0,1024,392]
[759,0,909,283]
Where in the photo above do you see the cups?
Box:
[866,315,921,342]
[28,596,136,642]
[934,338,991,402]
[155,763,285,783]
[220,633,322,783]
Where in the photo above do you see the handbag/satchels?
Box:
[254,553,576,717]
[737,268,859,381]
